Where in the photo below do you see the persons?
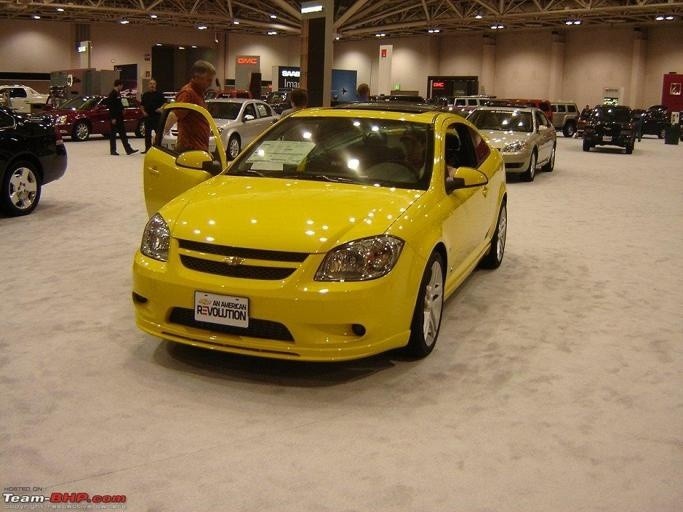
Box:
[140,79,168,154]
[352,83,369,103]
[164,59,216,153]
[580,104,591,121]
[107,79,139,155]
[281,88,309,172]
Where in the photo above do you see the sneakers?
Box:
[141,151,146,154]
[126,149,139,155]
[111,152,119,155]
